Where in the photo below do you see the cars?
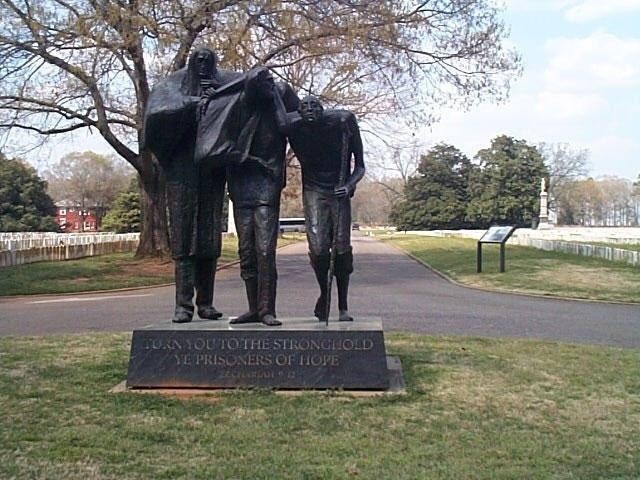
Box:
[352,223,359,230]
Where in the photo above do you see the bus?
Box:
[278,216,310,233]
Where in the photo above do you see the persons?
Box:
[144,48,232,322]
[280,91,366,322]
[193,67,295,326]
